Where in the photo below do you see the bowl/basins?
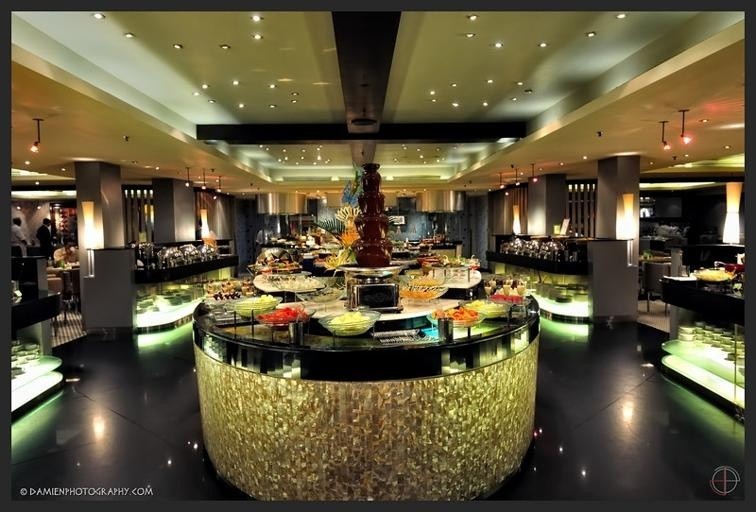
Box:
[225,267,529,337]
[678,320,744,371]
[694,267,735,281]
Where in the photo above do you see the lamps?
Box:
[512,205,521,235]
[679,108,691,145]
[660,121,671,151]
[81,200,95,249]
[30,119,48,151]
[185,167,222,194]
[201,208,209,238]
[622,193,634,241]
[721,182,743,246]
[497,163,537,189]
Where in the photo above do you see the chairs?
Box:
[56,270,72,326]
[642,261,672,317]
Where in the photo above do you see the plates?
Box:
[135,273,208,317]
[534,282,588,302]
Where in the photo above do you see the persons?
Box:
[12,216,28,255]
[53,239,79,263]
[196,218,202,241]
[36,217,52,255]
[255,221,273,245]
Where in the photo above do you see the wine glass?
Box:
[442,256,480,280]
